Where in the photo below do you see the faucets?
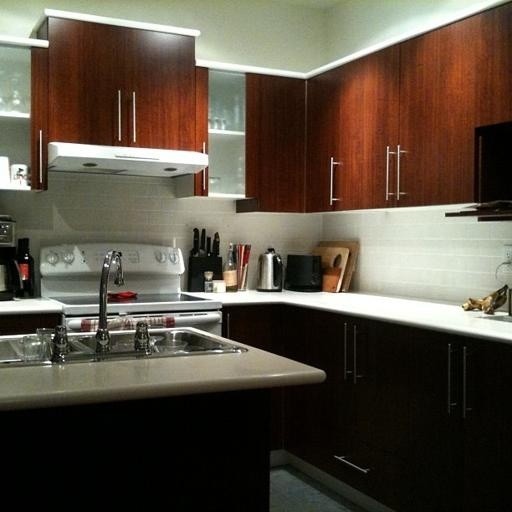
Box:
[96,250,123,351]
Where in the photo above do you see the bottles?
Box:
[17,238,34,296]
[203,271,213,292]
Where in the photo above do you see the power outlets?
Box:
[500,244,512,274]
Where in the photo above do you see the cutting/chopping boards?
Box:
[313,242,361,293]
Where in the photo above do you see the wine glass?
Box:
[0,71,27,115]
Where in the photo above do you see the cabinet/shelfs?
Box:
[31,37,50,192]
[1,44,29,190]
[209,69,246,198]
[306,62,342,213]
[344,1,492,211]
[50,8,202,190]
[195,59,209,195]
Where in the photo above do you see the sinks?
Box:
[76,330,249,358]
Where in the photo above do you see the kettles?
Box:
[255,247,284,291]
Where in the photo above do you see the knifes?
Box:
[190,227,220,257]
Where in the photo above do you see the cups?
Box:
[0,155,28,185]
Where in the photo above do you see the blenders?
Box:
[1,213,22,302]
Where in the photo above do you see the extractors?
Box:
[46,141,210,179]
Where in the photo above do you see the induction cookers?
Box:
[40,289,222,314]
